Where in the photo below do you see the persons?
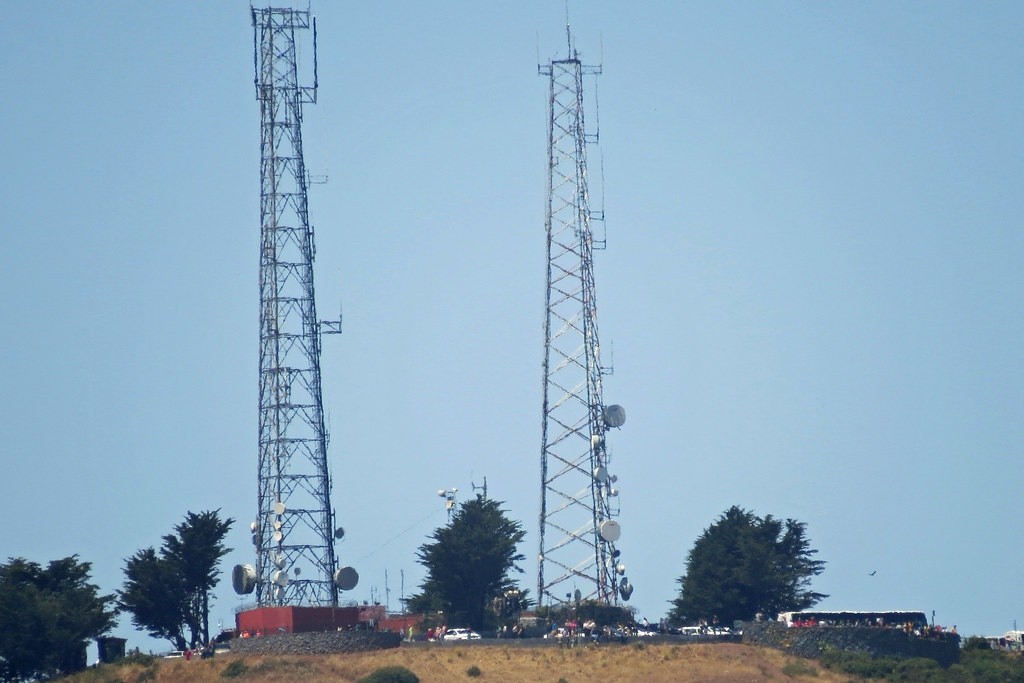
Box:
[659,617,711,635]
[408,624,417,641]
[348,623,353,630]
[337,624,343,633]
[400,627,405,640]
[547,618,636,645]
[793,615,958,637]
[496,621,525,639]
[424,625,448,640]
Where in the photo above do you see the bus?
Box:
[777,611,928,628]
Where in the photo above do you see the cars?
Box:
[444,628,481,640]
[681,625,743,635]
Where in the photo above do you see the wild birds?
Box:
[868,571,876,576]
